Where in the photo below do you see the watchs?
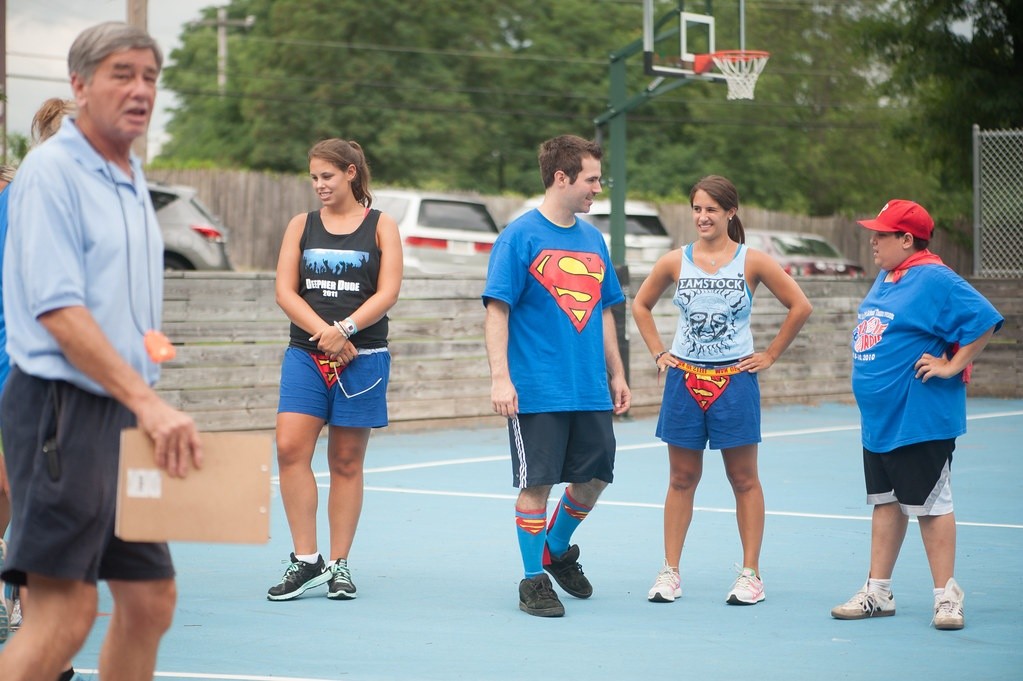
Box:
[342,320,354,335]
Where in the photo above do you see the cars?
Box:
[738,226,866,280]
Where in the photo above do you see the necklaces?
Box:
[701,241,729,266]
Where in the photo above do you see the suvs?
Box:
[510,193,675,282]
[144,178,236,273]
[364,188,504,279]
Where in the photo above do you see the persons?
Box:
[632,174,814,606]
[0,20,206,681]
[482,135,632,617]
[266,138,402,600]
[830,199,1004,629]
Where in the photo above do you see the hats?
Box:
[858,200,935,240]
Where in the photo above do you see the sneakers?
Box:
[543,541,593,599]
[930,578,965,629]
[267,552,333,600]
[648,558,682,601]
[831,571,895,619]
[327,558,357,600]
[726,563,766,604]
[519,574,565,616]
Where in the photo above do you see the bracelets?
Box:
[334,320,350,339]
[655,351,666,384]
[345,317,358,335]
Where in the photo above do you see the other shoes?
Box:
[69,672,93,681]
[1,539,16,644]
